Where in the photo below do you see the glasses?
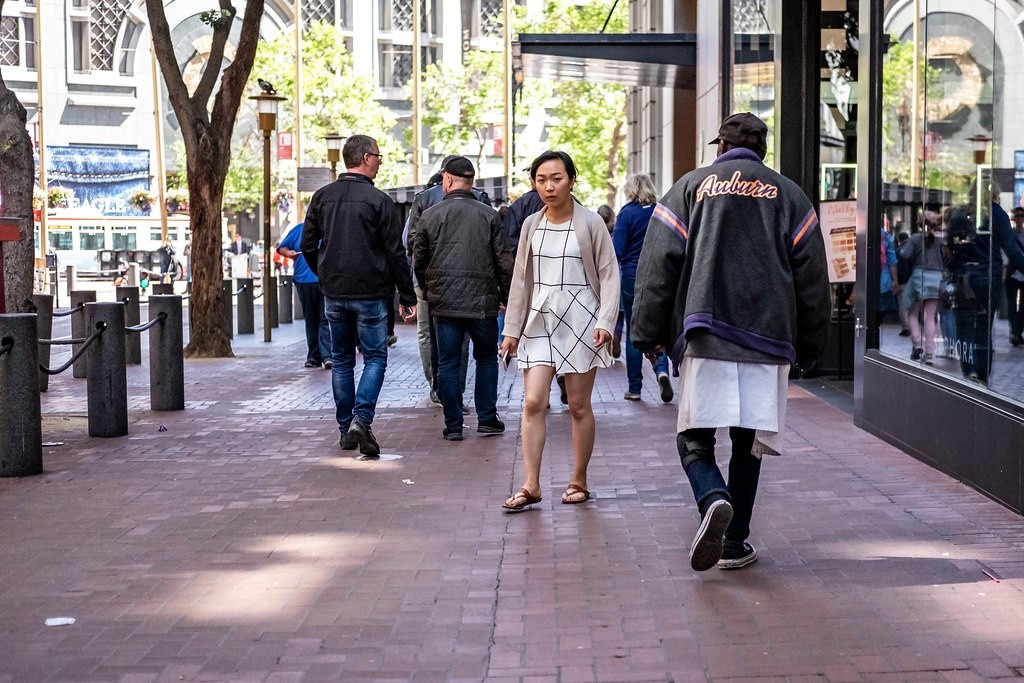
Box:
[363,153,382,161]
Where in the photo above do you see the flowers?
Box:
[128,191,156,212]
[271,191,296,211]
[164,195,191,213]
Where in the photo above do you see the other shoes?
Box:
[657,372,674,402]
[624,391,640,400]
[461,404,470,414]
[899,327,1024,389]
[305,360,321,367]
[672,371,679,377]
[324,360,333,369]
[430,390,439,404]
[387,335,398,346]
[556,373,568,404]
[613,336,621,358]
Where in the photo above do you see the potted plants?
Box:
[33,184,45,213]
[52,186,74,208]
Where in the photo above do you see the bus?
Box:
[29,215,193,286]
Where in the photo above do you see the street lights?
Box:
[246,91,288,343]
[321,132,348,184]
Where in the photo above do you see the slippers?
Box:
[502,488,542,509]
[561,484,590,504]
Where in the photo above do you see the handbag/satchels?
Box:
[895,253,919,284]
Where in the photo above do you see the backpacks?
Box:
[880,230,888,268]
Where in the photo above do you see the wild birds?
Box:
[257,78,277,94]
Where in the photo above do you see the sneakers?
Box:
[348,416,381,455]
[443,428,463,441]
[718,542,757,570]
[476,413,505,435]
[690,491,734,572]
[340,436,358,450]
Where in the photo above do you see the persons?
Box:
[879,175,1024,382]
[230,231,247,255]
[501,149,621,509]
[156,238,176,273]
[628,111,832,571]
[299,134,417,457]
[597,206,625,358]
[182,231,192,294]
[611,174,674,403]
[275,155,568,439]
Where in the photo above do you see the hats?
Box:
[707,112,767,149]
[438,155,459,174]
[439,157,475,178]
